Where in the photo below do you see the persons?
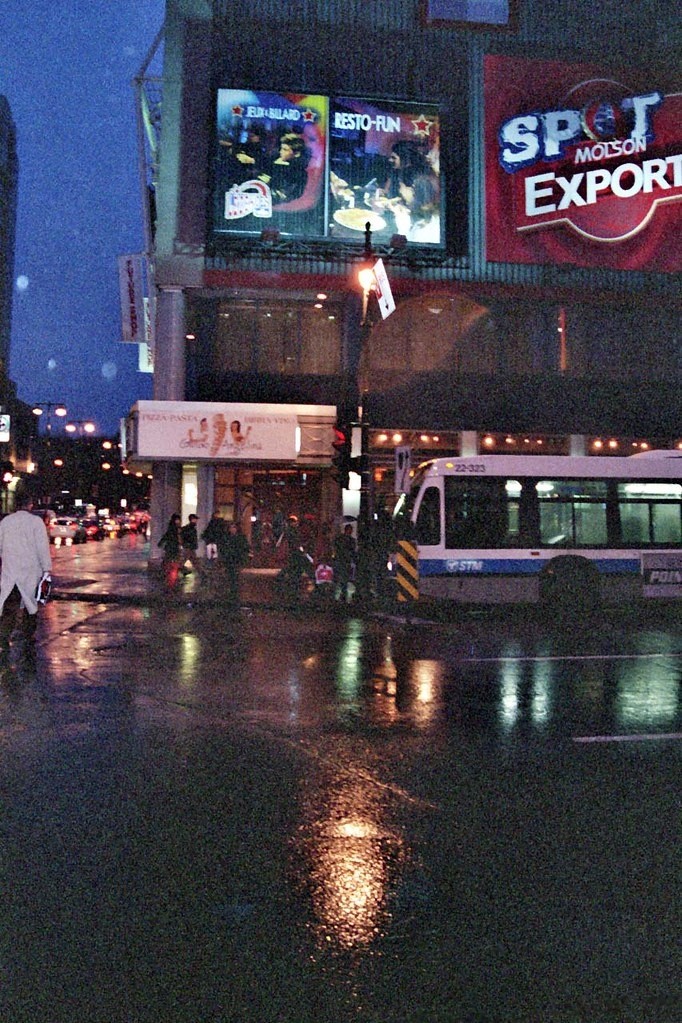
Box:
[260,509,360,600]
[384,117,439,244]
[217,122,307,206]
[0,492,53,674]
[157,510,253,595]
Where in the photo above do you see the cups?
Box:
[372,197,385,216]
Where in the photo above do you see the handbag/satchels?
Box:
[158,533,166,547]
[316,562,334,585]
[36,575,53,605]
[206,543,218,559]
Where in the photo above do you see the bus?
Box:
[386,449,682,616]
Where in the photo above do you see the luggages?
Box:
[163,556,186,595]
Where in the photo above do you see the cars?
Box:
[36,507,149,543]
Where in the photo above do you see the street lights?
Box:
[32,401,66,504]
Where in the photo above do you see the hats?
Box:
[189,514,201,521]
[285,513,299,521]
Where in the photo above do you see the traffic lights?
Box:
[328,423,350,490]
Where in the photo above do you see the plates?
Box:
[332,209,387,231]
